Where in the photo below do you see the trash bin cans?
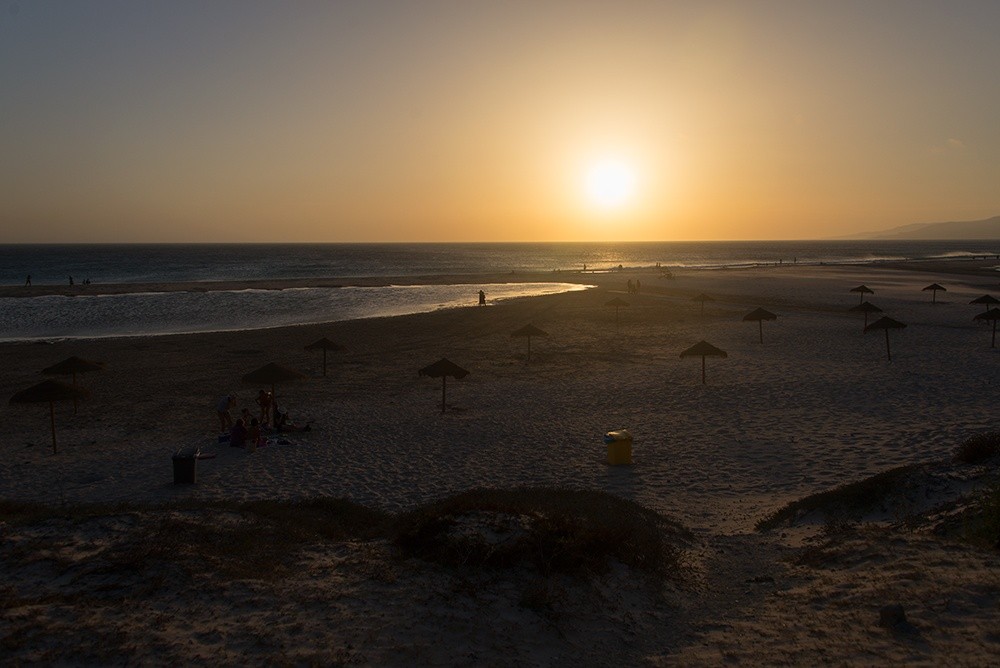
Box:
[171,447,200,485]
[605,428,634,465]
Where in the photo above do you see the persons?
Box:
[218,388,289,440]
[69,274,74,286]
[478,290,486,306]
[626,279,641,295]
[24,274,32,286]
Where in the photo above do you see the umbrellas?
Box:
[421,357,470,411]
[969,293,1000,349]
[508,324,549,360]
[864,315,908,362]
[744,308,777,342]
[921,282,948,297]
[240,361,305,400]
[851,284,884,322]
[305,337,343,372]
[604,297,629,318]
[692,291,717,314]
[681,340,728,382]
[10,355,114,454]
